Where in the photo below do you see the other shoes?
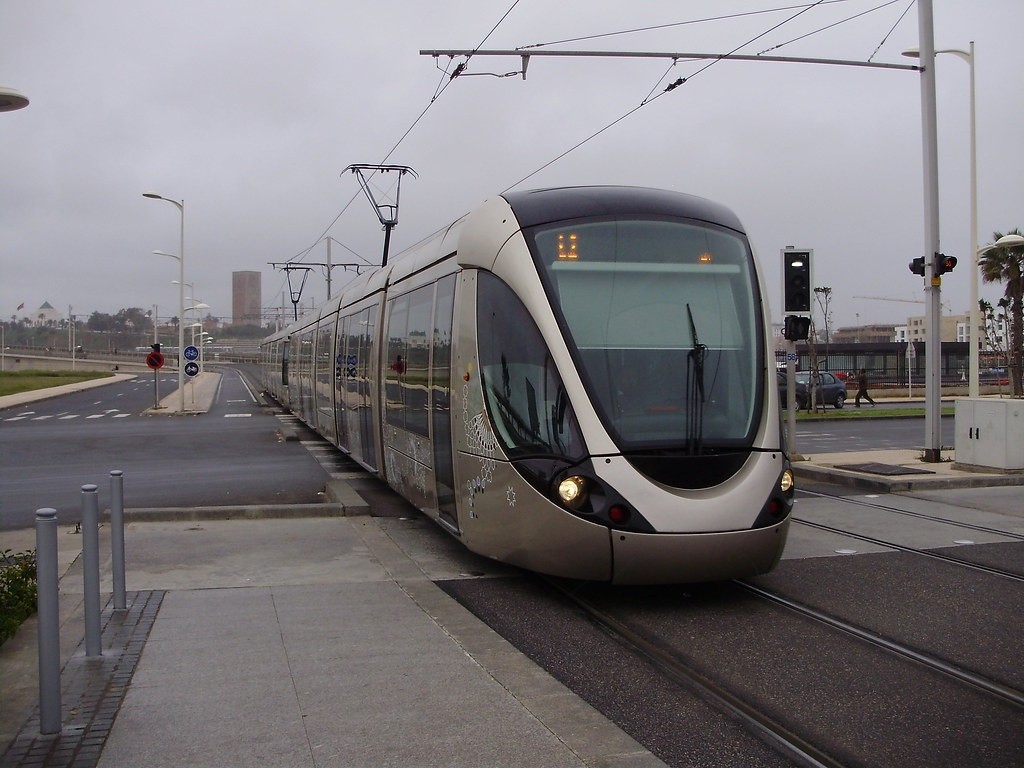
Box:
[871,403,876,407]
[854,405,860,407]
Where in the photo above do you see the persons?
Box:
[853,368,876,407]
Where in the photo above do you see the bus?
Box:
[136,346,234,355]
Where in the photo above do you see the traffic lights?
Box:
[935,252,958,275]
[150,344,160,354]
[784,315,814,343]
[780,248,813,314]
[906,256,925,276]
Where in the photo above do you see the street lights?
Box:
[65,320,75,370]
[142,191,189,417]
[904,38,1024,398]
[183,298,215,374]
[170,280,197,404]
[996,297,1014,397]
[0,325,10,372]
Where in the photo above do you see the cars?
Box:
[775,371,807,411]
[795,371,848,410]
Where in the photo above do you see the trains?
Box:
[258,164,795,591]
